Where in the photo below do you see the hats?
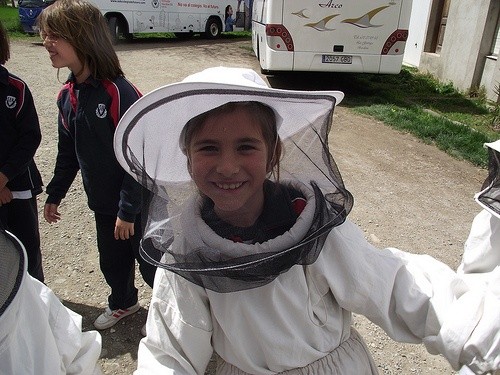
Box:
[112,66,346,183]
[484,140,500,153]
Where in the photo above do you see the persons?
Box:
[37,0,173,336]
[112,66,500,375]
[0,25,46,286]
[0,226,108,375]
[457,139,500,274]
[223,5,237,33]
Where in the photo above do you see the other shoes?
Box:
[141,325,146,336]
[94,302,140,329]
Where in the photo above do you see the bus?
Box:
[18,0,55,35]
[252,0,412,76]
[87,0,252,42]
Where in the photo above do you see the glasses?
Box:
[41,34,57,40]
